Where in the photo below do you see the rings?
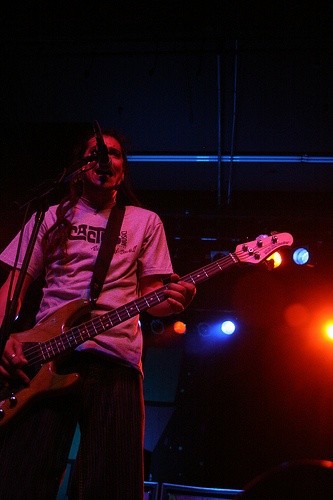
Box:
[8,354,16,359]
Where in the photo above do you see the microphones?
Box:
[92,122,114,176]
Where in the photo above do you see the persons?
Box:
[1,133,197,500]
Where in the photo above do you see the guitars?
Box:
[1,233,296,424]
